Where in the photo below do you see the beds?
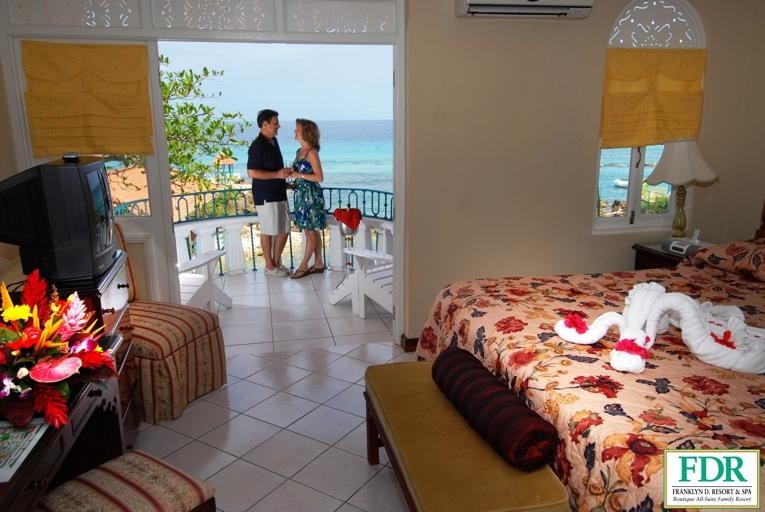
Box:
[414,201,765,512]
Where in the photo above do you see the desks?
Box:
[0,246,145,512]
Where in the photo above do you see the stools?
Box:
[44,451,218,512]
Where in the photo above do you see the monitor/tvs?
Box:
[0,156,118,290]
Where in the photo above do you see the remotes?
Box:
[62,151,81,160]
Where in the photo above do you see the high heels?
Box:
[290,269,309,279]
[309,265,324,274]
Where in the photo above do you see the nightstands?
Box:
[631,236,715,270]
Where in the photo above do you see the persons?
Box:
[289,119,327,279]
[246,109,294,278]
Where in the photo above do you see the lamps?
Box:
[643,132,719,249]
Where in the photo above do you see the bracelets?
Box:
[300,173,305,179]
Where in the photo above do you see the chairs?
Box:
[114,221,228,426]
[327,245,396,319]
[177,247,233,316]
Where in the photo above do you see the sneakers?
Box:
[279,266,293,275]
[262,269,289,277]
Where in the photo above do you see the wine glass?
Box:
[285,162,295,185]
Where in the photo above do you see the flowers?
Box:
[612,336,651,360]
[0,266,120,429]
[563,310,588,334]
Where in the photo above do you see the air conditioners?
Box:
[452,0,597,19]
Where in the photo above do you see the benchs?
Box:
[361,361,573,512]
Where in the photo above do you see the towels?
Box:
[555,277,765,376]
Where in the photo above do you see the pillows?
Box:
[430,346,560,472]
